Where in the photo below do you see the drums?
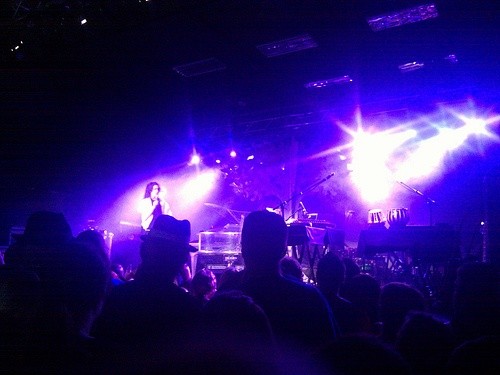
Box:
[199,232,241,254]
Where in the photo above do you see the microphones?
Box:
[321,172,335,183]
[300,201,306,212]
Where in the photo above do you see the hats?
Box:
[141,214,198,254]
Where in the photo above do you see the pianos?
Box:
[290,219,336,228]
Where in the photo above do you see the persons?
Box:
[140,181,173,238]
[0,208,500,375]
